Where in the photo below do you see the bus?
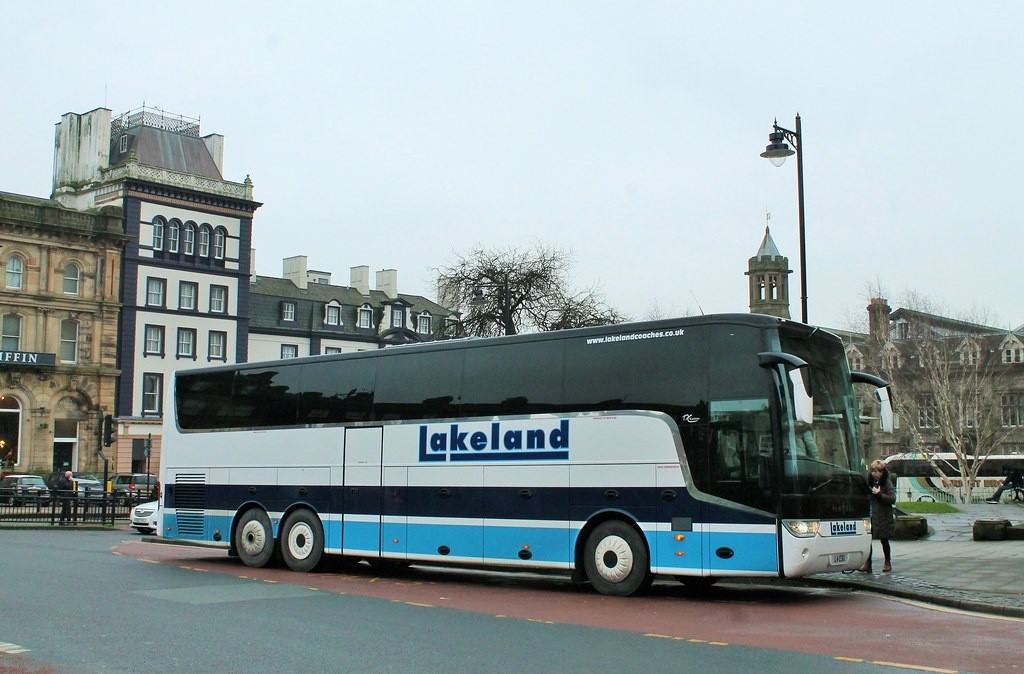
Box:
[882,451,1024,503]
[156,313,903,598]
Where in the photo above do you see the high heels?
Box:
[858,556,872,573]
[882,560,892,571]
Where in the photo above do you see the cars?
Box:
[0,474,50,507]
[48,470,111,506]
[128,500,158,534]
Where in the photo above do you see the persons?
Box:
[784,409,820,460]
[986,466,1024,504]
[148,482,159,503]
[59,471,74,526]
[857,460,896,573]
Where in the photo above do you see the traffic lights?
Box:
[103,414,118,446]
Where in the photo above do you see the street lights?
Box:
[758,120,808,325]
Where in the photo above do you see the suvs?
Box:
[108,471,159,506]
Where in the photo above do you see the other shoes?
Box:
[59,522,67,525]
[67,523,73,525]
